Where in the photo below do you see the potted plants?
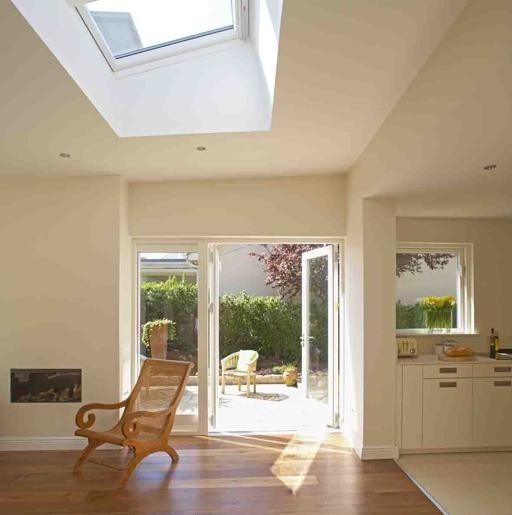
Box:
[283,367,298,386]
[145,320,175,360]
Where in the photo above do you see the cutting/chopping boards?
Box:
[446,356,476,362]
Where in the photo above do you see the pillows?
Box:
[237,350,256,373]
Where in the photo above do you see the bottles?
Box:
[495,329,500,352]
[490,328,496,358]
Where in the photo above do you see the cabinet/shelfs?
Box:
[397,355,512,454]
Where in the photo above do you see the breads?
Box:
[447,346,474,356]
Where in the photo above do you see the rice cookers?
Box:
[396,338,418,358]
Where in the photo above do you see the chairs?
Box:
[74,359,195,489]
[221,350,259,397]
